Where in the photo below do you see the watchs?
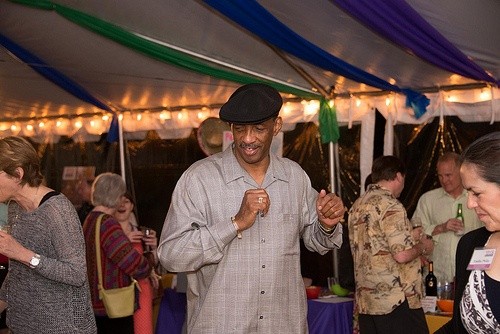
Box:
[320,224,336,234]
[29,253,41,270]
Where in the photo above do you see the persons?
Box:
[0,136,98,334]
[78,172,187,334]
[413,151,483,278]
[453,132,500,334]
[348,155,435,334]
[187,116,224,161]
[157,83,346,334]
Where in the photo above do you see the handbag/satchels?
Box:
[98,281,135,319]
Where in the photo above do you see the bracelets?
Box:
[232,215,242,239]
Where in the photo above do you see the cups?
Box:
[410,217,422,230]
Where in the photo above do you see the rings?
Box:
[259,197,263,204]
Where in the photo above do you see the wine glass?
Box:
[0,225,12,269]
[141,227,153,253]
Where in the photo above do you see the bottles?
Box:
[425,261,437,296]
[437,281,449,295]
[456,204,465,236]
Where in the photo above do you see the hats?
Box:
[198,115,232,154]
[219,82,284,126]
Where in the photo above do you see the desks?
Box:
[155,287,354,334]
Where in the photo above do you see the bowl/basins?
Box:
[437,300,454,312]
[327,277,349,297]
[305,286,321,299]
[303,278,312,286]
